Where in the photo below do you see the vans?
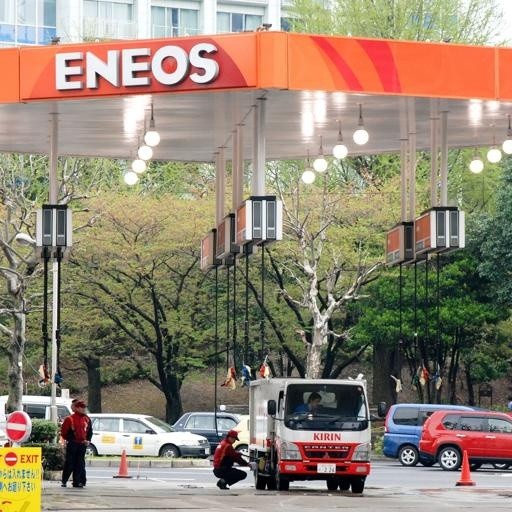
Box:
[382,403,492,470]
[1,394,90,423]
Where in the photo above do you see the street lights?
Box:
[16,233,60,445]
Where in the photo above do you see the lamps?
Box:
[331,119,349,161]
[500,111,512,156]
[353,100,371,148]
[485,119,503,165]
[313,133,329,174]
[301,147,316,187]
[467,125,485,175]
[129,101,161,174]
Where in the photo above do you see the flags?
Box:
[219,354,272,392]
[392,363,443,392]
[38,361,64,389]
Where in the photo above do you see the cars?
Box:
[418,409,512,470]
[170,411,242,450]
[230,414,250,465]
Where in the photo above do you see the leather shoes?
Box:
[217,480,229,490]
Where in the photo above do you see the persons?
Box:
[60,400,90,487]
[214,430,257,490]
[294,392,322,414]
[70,399,93,487]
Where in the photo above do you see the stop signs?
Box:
[4,411,32,443]
[0,447,41,512]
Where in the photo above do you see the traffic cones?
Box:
[455,449,476,486]
[112,449,133,478]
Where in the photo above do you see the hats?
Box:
[228,430,239,441]
[74,400,87,408]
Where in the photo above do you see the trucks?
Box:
[248,378,387,494]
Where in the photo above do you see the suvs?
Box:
[71,412,210,458]
